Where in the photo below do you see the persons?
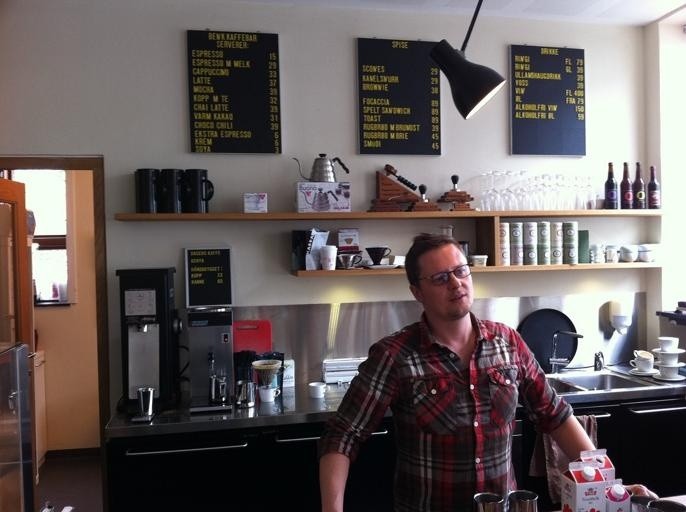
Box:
[316,231,659,512]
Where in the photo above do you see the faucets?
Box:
[549,331,583,374]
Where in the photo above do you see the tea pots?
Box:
[292,154,349,183]
[301,188,338,211]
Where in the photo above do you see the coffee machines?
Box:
[186,302,234,413]
[115,267,185,422]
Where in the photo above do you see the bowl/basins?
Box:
[620,244,653,262]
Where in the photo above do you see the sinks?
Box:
[546,376,580,393]
[567,375,645,391]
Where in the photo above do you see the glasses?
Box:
[416,263,473,287]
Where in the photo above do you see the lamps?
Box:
[426,0,508,122]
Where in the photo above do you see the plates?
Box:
[628,370,660,376]
[654,361,686,367]
[651,348,686,354]
[365,265,398,269]
[632,369,659,374]
[652,372,685,381]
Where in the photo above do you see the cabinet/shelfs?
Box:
[101,424,321,512]
[108,207,662,279]
[507,401,624,512]
[269,416,417,512]
[29,350,48,476]
[617,398,686,498]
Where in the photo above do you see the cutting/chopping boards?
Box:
[232,320,272,400]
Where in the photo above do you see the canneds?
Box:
[550,221,563,244]
[510,244,523,265]
[563,222,578,243]
[523,222,537,245]
[538,244,550,265]
[523,245,538,265]
[563,243,579,264]
[500,245,510,266]
[499,222,510,245]
[510,222,523,244]
[538,221,550,245]
[550,244,563,265]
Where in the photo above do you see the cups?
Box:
[308,381,332,399]
[659,367,679,379]
[474,490,539,512]
[338,254,363,268]
[235,378,280,409]
[657,337,679,352]
[629,358,654,372]
[631,494,686,512]
[633,349,653,361]
[657,354,678,365]
[135,169,214,213]
[366,248,391,264]
[319,245,338,271]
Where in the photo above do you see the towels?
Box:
[529,415,598,504]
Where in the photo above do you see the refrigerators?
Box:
[0,342,36,512]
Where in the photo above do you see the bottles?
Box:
[605,161,661,208]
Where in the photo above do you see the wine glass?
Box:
[481,169,596,212]
